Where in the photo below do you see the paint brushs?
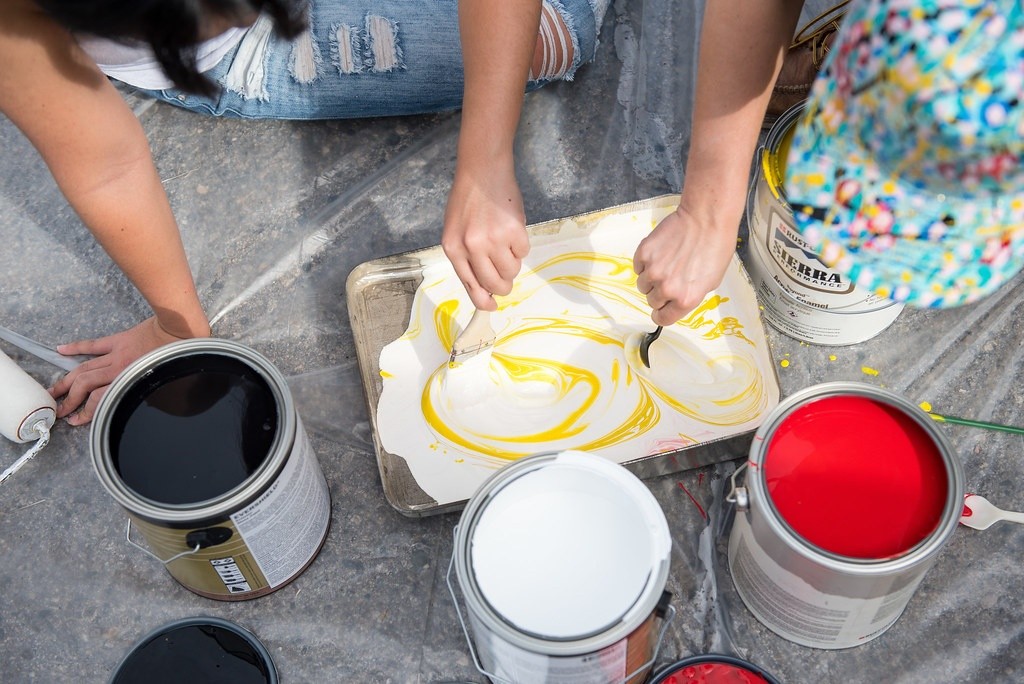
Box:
[928,412,1024,435]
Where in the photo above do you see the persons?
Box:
[0,1,616,426]
[632,1,1024,327]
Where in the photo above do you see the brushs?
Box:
[448,292,497,363]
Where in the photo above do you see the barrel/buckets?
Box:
[445,449,677,683]
[648,655,779,684]
[108,617,278,684]
[725,381,965,649]
[747,96,905,346]
[89,337,332,602]
